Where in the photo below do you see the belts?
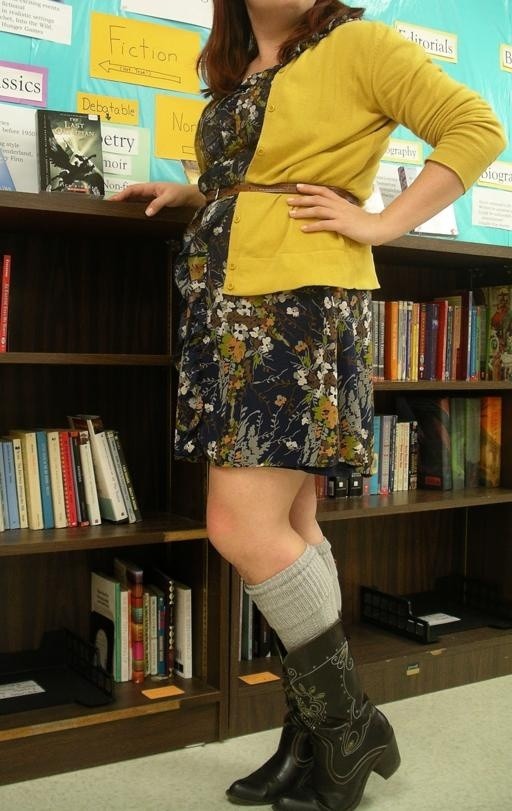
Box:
[205,183,364,206]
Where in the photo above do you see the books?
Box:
[236,573,275,662]
[1,423,197,719]
[1,257,14,354]
[316,285,512,503]
[39,106,104,197]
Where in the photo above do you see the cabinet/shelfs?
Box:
[0,187,511,789]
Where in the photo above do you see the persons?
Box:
[110,0,508,811]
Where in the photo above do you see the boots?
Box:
[227,611,342,805]
[273,618,401,811]
